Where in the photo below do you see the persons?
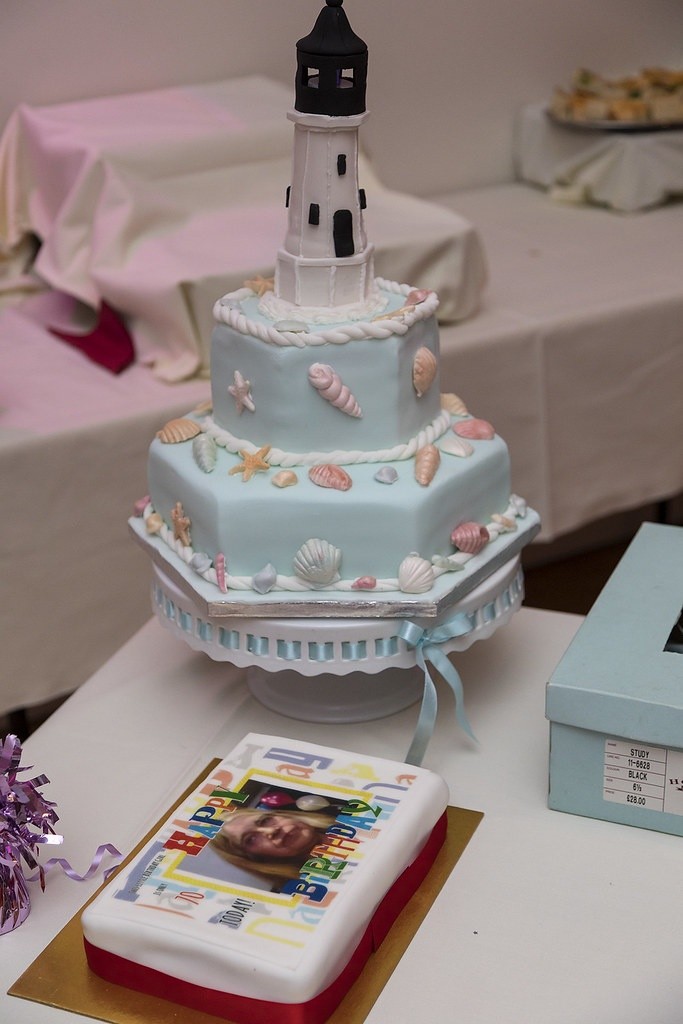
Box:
[211,807,337,878]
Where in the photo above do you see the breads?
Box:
[547,66,683,125]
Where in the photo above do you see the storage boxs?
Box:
[545,521,683,838]
[84,728,449,1024]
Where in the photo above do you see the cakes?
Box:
[134,0,527,595]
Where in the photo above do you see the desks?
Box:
[0,608,683,1024]
[0,184,683,718]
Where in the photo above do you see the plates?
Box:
[546,109,683,131]
